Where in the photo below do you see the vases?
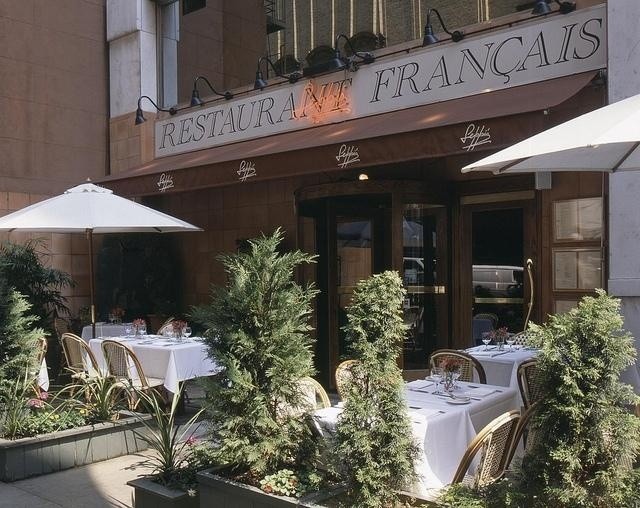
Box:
[497,336,504,351]
[114,316,121,324]
[136,327,142,338]
[175,331,183,342]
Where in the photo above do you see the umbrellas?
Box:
[337,220,437,247]
[461,94,640,176]
[0,178,204,338]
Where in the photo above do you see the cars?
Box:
[404,257,437,286]
[470,264,525,323]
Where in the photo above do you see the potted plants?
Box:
[0,277,153,483]
[194,222,453,508]
[94,370,219,508]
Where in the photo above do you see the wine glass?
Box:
[107,313,194,347]
[427,328,520,405]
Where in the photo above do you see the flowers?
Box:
[173,320,186,332]
[112,307,122,316]
[490,326,509,342]
[132,319,145,327]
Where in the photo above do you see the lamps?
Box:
[189,75,233,107]
[259,1,285,34]
[532,1,577,16]
[254,55,300,90]
[328,34,375,71]
[421,8,464,47]
[134,96,178,125]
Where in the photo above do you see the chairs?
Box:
[157,321,187,337]
[24,317,105,400]
[101,340,170,423]
[427,349,553,490]
[403,305,425,352]
[334,360,362,402]
[273,375,332,428]
